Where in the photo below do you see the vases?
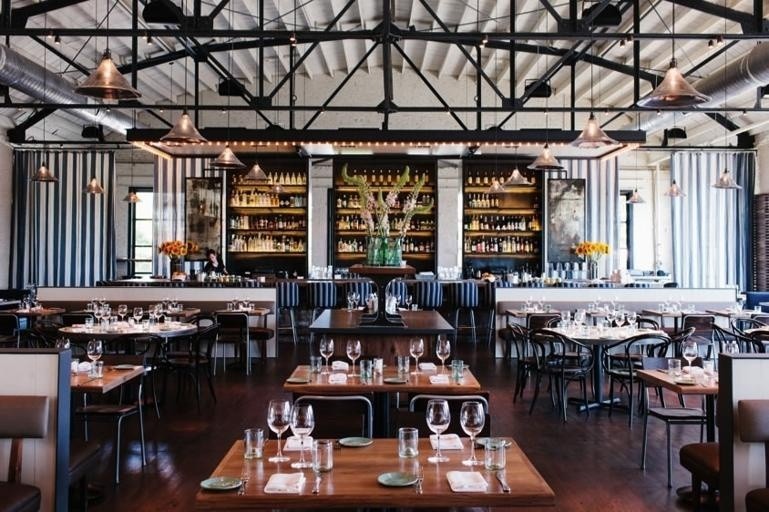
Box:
[366,236,385,265]
[591,262,598,280]
[170,262,182,280]
[386,236,402,266]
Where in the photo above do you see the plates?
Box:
[338,437,374,447]
[447,363,469,369]
[199,476,242,491]
[378,471,418,488]
[114,365,133,371]
[414,274,436,280]
[372,363,386,369]
[287,377,308,384]
[674,378,698,388]
[383,377,409,384]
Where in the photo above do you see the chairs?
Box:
[0,267,769,511]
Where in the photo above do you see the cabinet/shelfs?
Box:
[463,186,540,260]
[333,185,436,262]
[228,184,307,260]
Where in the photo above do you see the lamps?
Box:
[485,0,740,204]
[28,0,268,203]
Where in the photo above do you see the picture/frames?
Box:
[547,178,586,263]
[184,177,223,262]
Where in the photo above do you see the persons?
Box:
[203,249,230,275]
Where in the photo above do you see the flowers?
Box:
[160,241,187,262]
[575,241,609,262]
[341,162,435,266]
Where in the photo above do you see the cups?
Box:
[720,342,738,354]
[313,439,334,473]
[699,358,715,375]
[732,301,761,315]
[56,337,106,380]
[412,304,418,312]
[483,437,507,470]
[451,359,465,379]
[250,303,254,311]
[397,355,410,374]
[243,428,264,460]
[177,304,183,312]
[84,317,94,329]
[374,357,384,376]
[547,261,587,281]
[667,360,681,377]
[360,359,372,380]
[87,303,92,310]
[398,427,419,459]
[311,357,322,373]
[227,302,232,311]
[197,271,242,283]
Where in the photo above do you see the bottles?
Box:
[461,167,542,281]
[229,167,434,253]
[335,267,350,280]
[283,268,298,280]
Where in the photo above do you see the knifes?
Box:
[309,471,322,494]
[496,470,512,492]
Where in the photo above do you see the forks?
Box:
[240,468,250,496]
[416,466,424,496]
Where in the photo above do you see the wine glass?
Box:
[408,337,424,375]
[678,339,697,375]
[266,397,292,464]
[519,295,696,338]
[346,291,412,312]
[320,339,335,375]
[23,293,39,310]
[288,402,314,469]
[230,294,250,312]
[435,337,451,373]
[425,399,451,464]
[460,401,485,467]
[346,341,362,378]
[91,297,177,329]
